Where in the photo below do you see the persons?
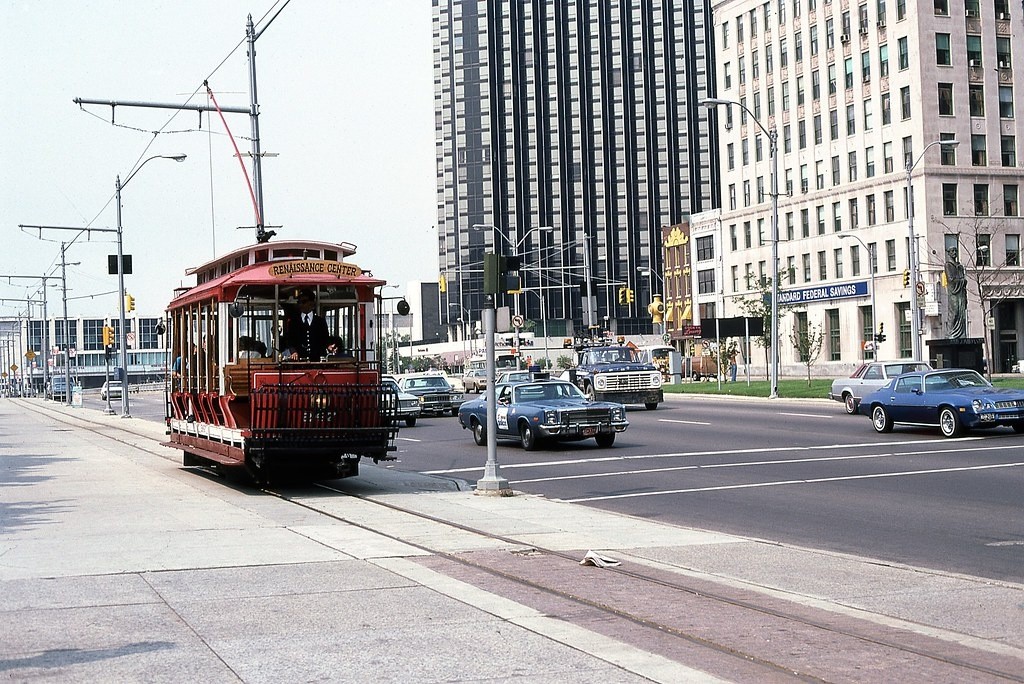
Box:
[526,340,530,345]
[271,325,291,356]
[497,389,522,404]
[945,246,968,338]
[328,336,351,358]
[521,358,524,363]
[526,355,532,366]
[543,385,564,399]
[731,352,737,381]
[409,381,415,387]
[172,342,197,391]
[284,290,338,362]
[615,350,628,361]
[193,334,212,391]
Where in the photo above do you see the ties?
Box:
[305,316,309,327]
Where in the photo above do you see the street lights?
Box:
[521,289,549,370]
[472,223,553,380]
[700,98,778,399]
[637,267,666,344]
[838,235,877,361]
[904,140,959,371]
[963,245,988,339]
[457,318,467,368]
[116,154,188,419]
[449,303,472,357]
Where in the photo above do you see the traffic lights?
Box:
[619,288,634,304]
[103,327,115,346]
[904,269,910,288]
[439,275,446,292]
[879,322,883,333]
[127,295,135,312]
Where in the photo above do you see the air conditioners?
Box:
[966,10,976,17]
[970,60,982,67]
[725,123,732,129]
[877,20,885,28]
[1000,12,1011,20]
[1000,61,1011,68]
[859,27,867,35]
[840,34,849,44]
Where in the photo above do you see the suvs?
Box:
[570,346,664,411]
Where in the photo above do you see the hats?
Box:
[297,289,315,299]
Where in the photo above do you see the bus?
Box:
[467,355,516,369]
[155,76,400,488]
[600,346,676,372]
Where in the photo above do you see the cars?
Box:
[380,372,466,427]
[47,374,75,400]
[100,381,122,400]
[23,388,36,397]
[828,361,1024,439]
[461,369,629,451]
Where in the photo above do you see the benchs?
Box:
[170,358,370,429]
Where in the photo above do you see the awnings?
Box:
[663,308,673,321]
[681,305,691,319]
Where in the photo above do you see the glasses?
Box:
[297,299,311,305]
